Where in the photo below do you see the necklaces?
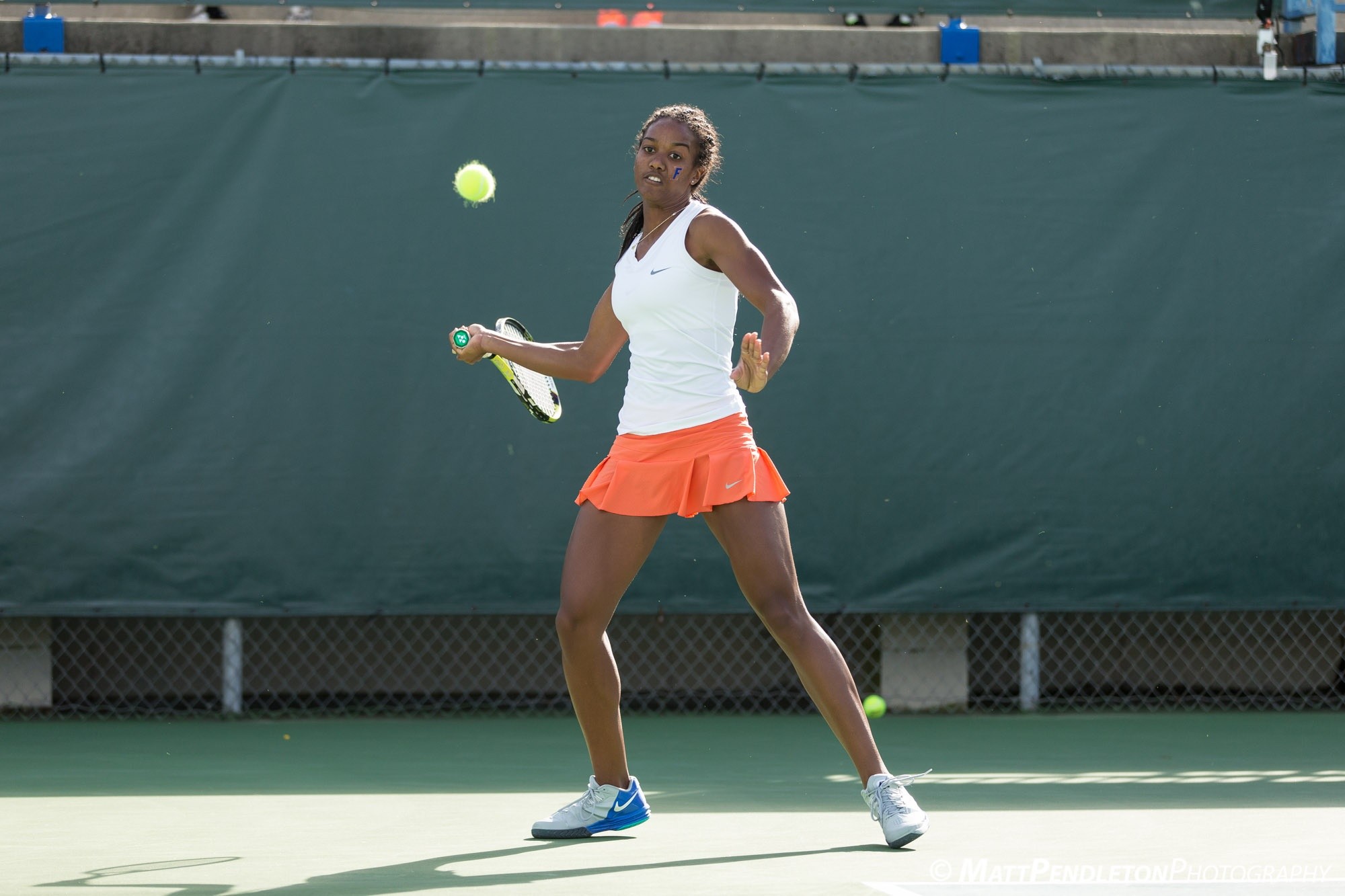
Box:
[632,205,689,250]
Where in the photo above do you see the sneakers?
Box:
[531,775,651,839]
[860,768,933,848]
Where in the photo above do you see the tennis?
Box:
[862,694,887,719]
[454,162,494,203]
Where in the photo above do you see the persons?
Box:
[447,103,934,847]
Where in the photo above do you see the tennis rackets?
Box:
[452,316,564,423]
